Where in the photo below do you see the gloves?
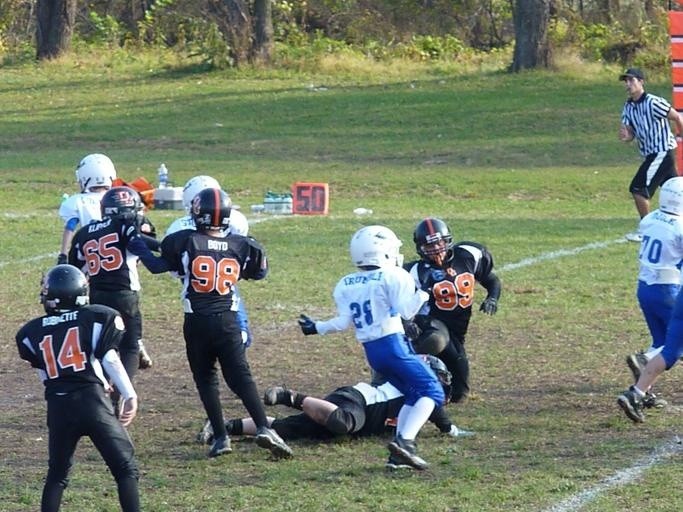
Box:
[57,254,67,265]
[123,219,141,246]
[441,425,478,438]
[479,296,497,315]
[403,320,422,340]
[297,314,318,335]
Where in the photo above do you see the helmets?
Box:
[417,354,454,406]
[182,176,220,215]
[39,264,89,315]
[190,189,232,232]
[350,225,404,269]
[100,187,145,221]
[75,153,117,194]
[659,176,683,216]
[413,219,454,269]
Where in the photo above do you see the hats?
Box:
[619,69,644,82]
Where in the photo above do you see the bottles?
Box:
[158,163,167,189]
[265,191,293,215]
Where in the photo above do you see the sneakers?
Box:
[264,384,298,408]
[626,232,642,243]
[197,418,233,444]
[641,391,667,410]
[385,454,414,479]
[627,350,648,386]
[388,432,429,470]
[204,436,232,458]
[254,427,293,461]
[617,387,645,423]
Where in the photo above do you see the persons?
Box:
[197,355,476,442]
[69,186,161,418]
[615,288,681,426]
[16,264,141,511]
[618,69,681,241]
[166,175,250,348]
[297,226,445,471]
[617,174,681,382]
[114,187,293,461]
[401,218,502,403]
[59,153,155,368]
